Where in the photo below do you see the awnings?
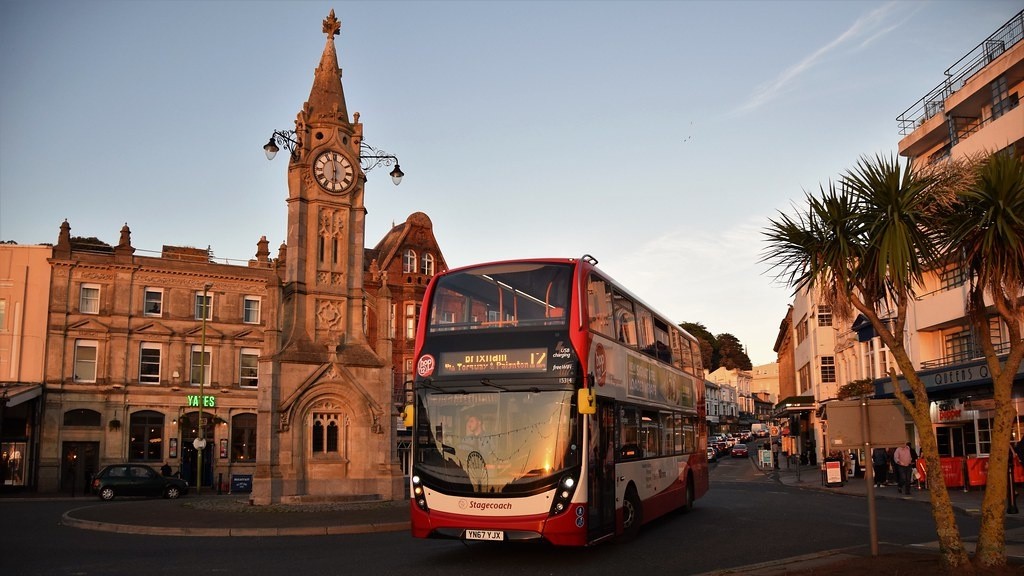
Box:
[873,351,1024,402]
[771,395,816,417]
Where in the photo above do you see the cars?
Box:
[707,423,769,462]
[731,444,749,458]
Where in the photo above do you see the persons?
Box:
[458,415,496,460]
[8,450,21,486]
[871,440,928,495]
[804,438,813,466]
[764,439,781,470]
[145,461,173,477]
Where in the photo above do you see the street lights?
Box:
[193,280,215,492]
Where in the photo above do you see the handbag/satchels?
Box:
[911,468,921,483]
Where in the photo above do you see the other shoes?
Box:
[898,488,902,493]
[905,492,910,495]
[874,484,877,488]
[880,485,887,487]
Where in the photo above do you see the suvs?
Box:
[91,463,191,501]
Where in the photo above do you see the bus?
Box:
[397,254,710,551]
